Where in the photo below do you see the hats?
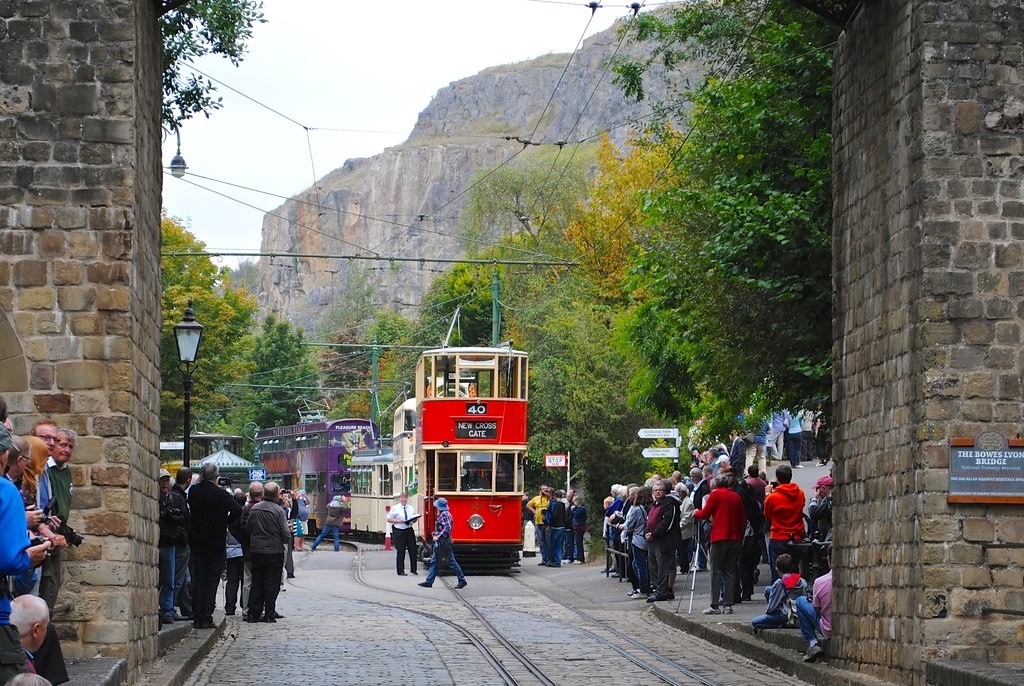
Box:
[159,469,171,478]
[715,455,730,464]
[0,421,20,452]
[433,498,449,511]
[333,496,341,501]
[812,476,833,488]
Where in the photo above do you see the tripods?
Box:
[675,517,711,615]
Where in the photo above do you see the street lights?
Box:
[173,300,204,468]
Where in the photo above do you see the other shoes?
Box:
[399,573,408,577]
[159,546,316,627]
[454,580,466,589]
[419,581,433,588]
[411,571,418,575]
[537,555,769,614]
[791,464,804,468]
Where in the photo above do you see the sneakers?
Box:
[802,644,824,661]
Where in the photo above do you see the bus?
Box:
[253,395,379,537]
[350,304,531,575]
[159,414,243,481]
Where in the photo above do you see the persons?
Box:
[601,401,833,662]
[158,462,311,631]
[0,395,77,686]
[417,497,467,589]
[387,492,422,576]
[310,495,350,552]
[522,484,586,567]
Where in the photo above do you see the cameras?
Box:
[218,478,231,486]
[55,521,85,548]
[37,513,48,523]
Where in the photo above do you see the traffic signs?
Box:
[637,428,681,439]
[641,447,680,458]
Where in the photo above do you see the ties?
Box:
[404,507,409,525]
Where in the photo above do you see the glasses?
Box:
[33,435,60,444]
[159,480,170,484]
[16,456,32,464]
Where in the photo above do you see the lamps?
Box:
[160,113,189,178]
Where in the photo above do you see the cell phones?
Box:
[771,482,779,488]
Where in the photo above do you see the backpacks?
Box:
[784,586,802,627]
[638,505,648,539]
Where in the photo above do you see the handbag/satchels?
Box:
[742,427,755,443]
[741,520,755,555]
